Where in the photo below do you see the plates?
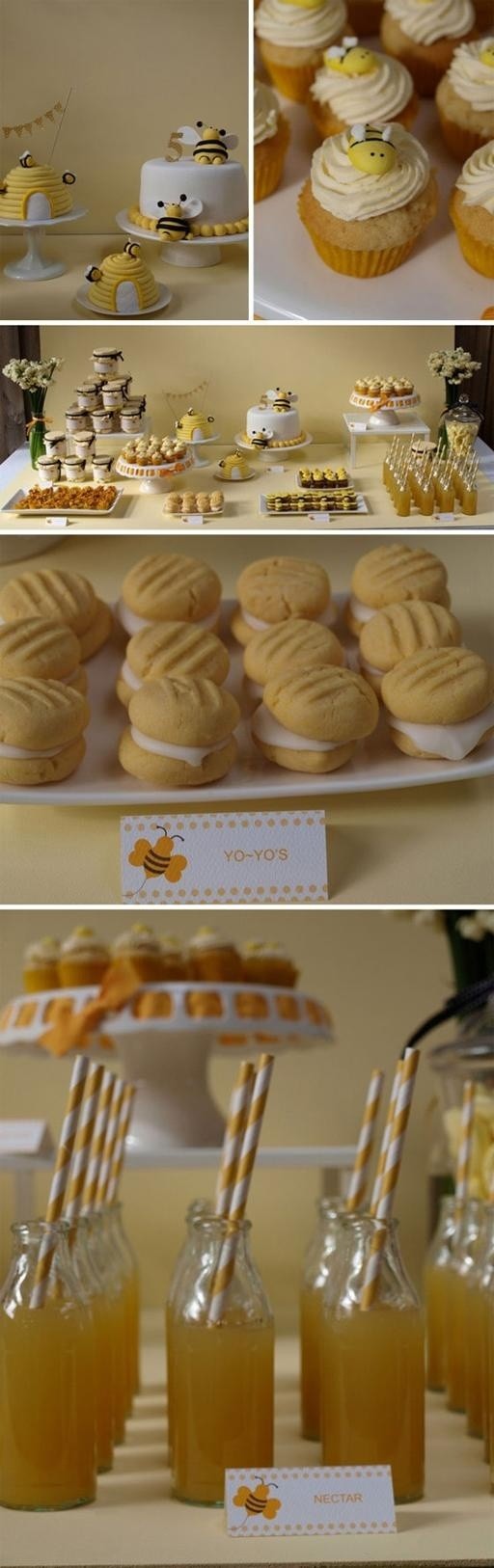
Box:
[296,472,356,490]
[74,277,173,315]
[0,482,124,517]
[257,494,373,515]
[162,496,227,517]
[2,586,490,808]
[213,469,256,482]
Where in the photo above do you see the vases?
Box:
[27,410,50,470]
[435,397,449,460]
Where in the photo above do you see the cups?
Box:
[443,393,481,457]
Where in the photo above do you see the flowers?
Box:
[1,353,64,461]
[427,344,483,413]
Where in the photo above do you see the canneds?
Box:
[37,456,60,482]
[43,431,67,459]
[73,431,92,460]
[63,455,86,484]
[64,347,145,433]
[91,455,113,483]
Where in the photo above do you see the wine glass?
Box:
[234,431,312,463]
[115,206,248,267]
[0,207,89,282]
[115,455,191,494]
[178,431,221,472]
[348,389,422,427]
[1,981,337,1147]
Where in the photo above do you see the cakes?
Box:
[22,922,298,994]
[174,407,215,442]
[0,151,75,222]
[354,374,414,398]
[1,547,494,788]
[217,450,251,480]
[266,489,357,511]
[127,120,249,241]
[80,239,158,313]
[165,490,224,513]
[254,1,494,280]
[299,467,348,488]
[122,433,186,465]
[239,387,306,449]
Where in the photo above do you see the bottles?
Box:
[382,433,481,516]
[1,1200,493,1513]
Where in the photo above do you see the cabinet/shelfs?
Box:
[0,427,493,530]
